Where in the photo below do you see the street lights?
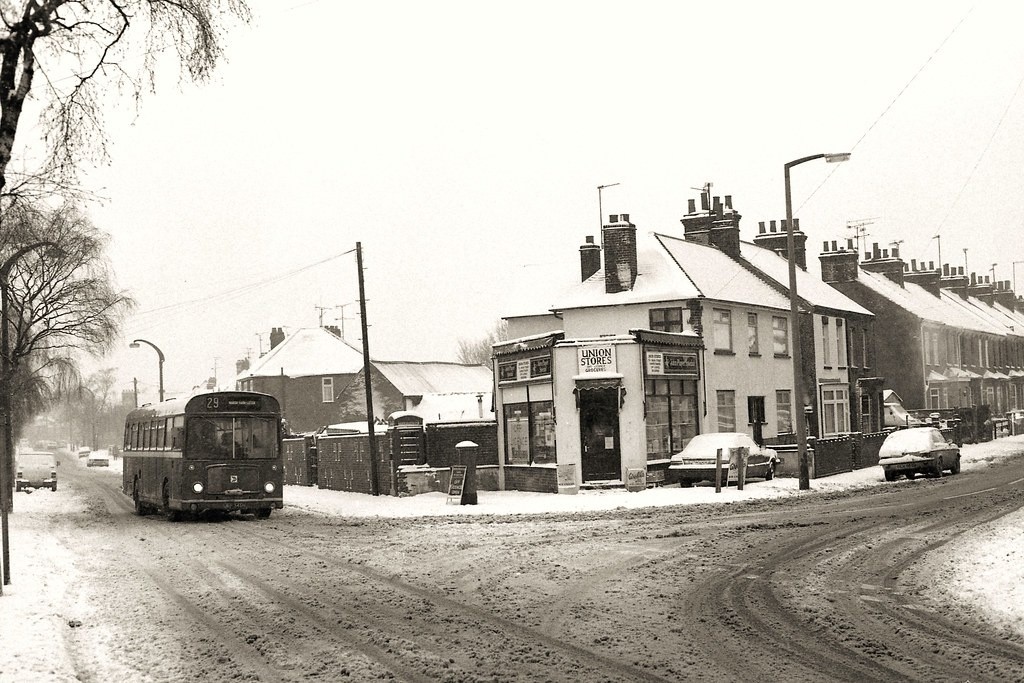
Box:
[0,241,74,586]
[129,339,165,402]
[963,249,968,276]
[932,235,941,268]
[784,154,851,488]
[1013,261,1024,293]
[989,263,997,282]
[597,182,620,250]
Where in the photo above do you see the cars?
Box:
[15,452,61,492]
[47,442,57,449]
[86,451,109,467]
[78,447,90,458]
[668,433,777,488]
[878,427,961,482]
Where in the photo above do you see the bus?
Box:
[121,391,288,522]
